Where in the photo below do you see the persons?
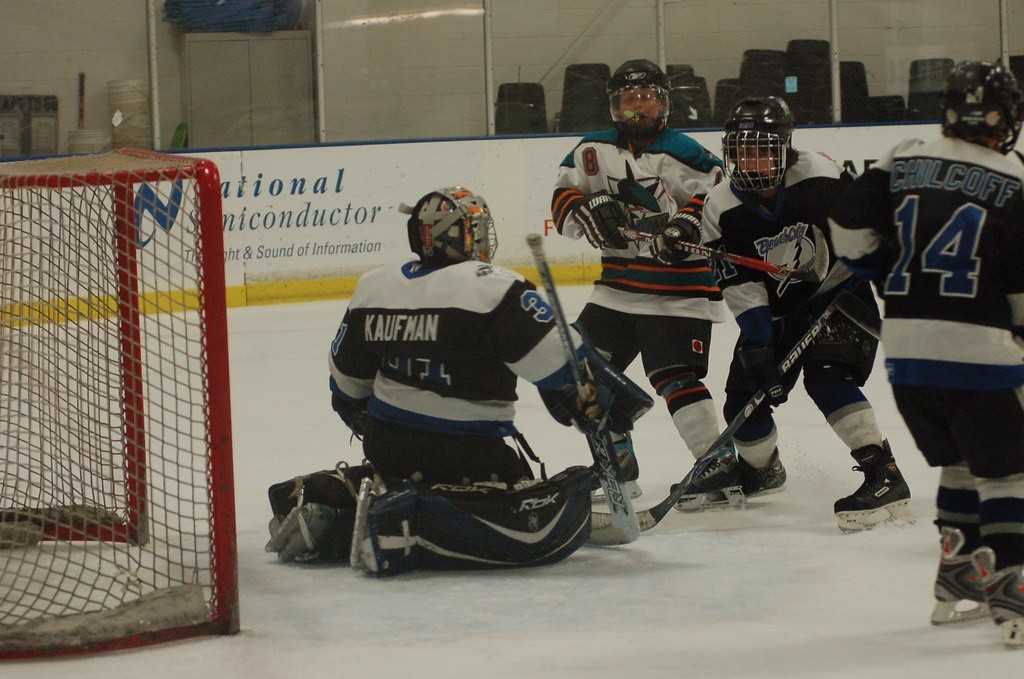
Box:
[264,188,655,575]
[548,61,726,502]
[829,59,1024,651]
[670,92,915,528]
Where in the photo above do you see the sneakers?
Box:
[349,477,387,570]
[587,429,641,502]
[267,502,335,561]
[929,527,992,624]
[970,546,1024,647]
[671,447,789,511]
[832,437,914,531]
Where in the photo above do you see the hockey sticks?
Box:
[618,225,828,283]
[833,288,882,340]
[525,234,642,544]
[589,274,864,532]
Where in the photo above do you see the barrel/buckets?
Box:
[67,128,112,154]
[106,79,153,152]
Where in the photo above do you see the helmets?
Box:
[407,185,499,263]
[722,93,797,193]
[940,58,1024,156]
[607,59,672,137]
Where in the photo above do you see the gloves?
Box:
[570,188,629,249]
[737,342,790,408]
[656,210,703,266]
